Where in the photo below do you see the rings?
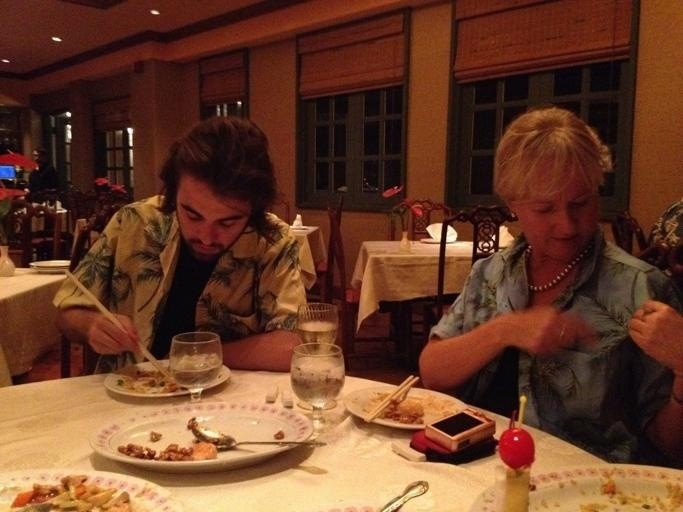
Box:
[560,330,566,337]
[640,308,653,320]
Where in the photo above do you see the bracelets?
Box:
[669,390,683,406]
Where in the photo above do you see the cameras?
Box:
[425,408,496,452]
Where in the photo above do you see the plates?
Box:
[342,386,469,432]
[104,359,231,399]
[0,468,185,512]
[420,238,441,244]
[88,401,313,475]
[28,260,71,273]
[467,463,683,511]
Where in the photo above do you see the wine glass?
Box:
[291,342,346,446]
[297,303,339,411]
[169,332,223,402]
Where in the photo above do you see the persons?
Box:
[27,146,60,193]
[49,115,311,374]
[418,108,683,465]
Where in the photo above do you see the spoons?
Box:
[192,427,327,451]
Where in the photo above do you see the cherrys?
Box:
[498,408,535,467]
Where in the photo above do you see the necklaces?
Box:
[525,244,591,292]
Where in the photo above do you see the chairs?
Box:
[422,206,519,346]
[10,225,100,381]
[390,199,453,242]
[610,211,648,258]
[325,200,412,371]
[306,193,345,302]
[0,185,128,267]
[266,193,290,227]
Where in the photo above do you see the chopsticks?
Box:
[62,268,172,383]
[365,374,420,423]
